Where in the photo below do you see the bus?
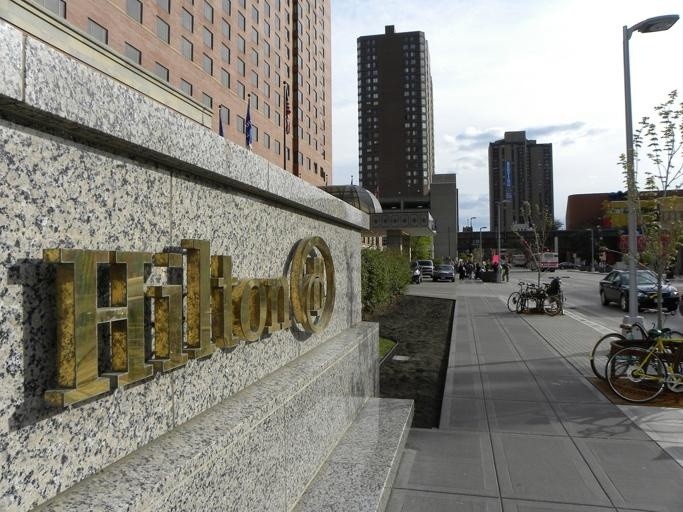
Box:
[529,252,559,272]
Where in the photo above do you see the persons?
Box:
[501,263,511,282]
[455,254,502,283]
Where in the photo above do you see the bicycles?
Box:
[505,275,570,316]
[588,316,683,403]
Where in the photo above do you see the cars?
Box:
[431,264,455,282]
[558,261,582,270]
[598,267,679,313]
[419,259,435,277]
[408,260,423,285]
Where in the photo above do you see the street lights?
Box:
[585,228,595,272]
[470,217,476,232]
[496,199,511,257]
[618,15,679,342]
[479,226,487,266]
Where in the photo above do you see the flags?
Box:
[283,83,292,135]
[246,94,252,153]
[218,105,225,138]
[350,175,355,208]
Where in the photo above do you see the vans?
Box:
[512,254,526,266]
